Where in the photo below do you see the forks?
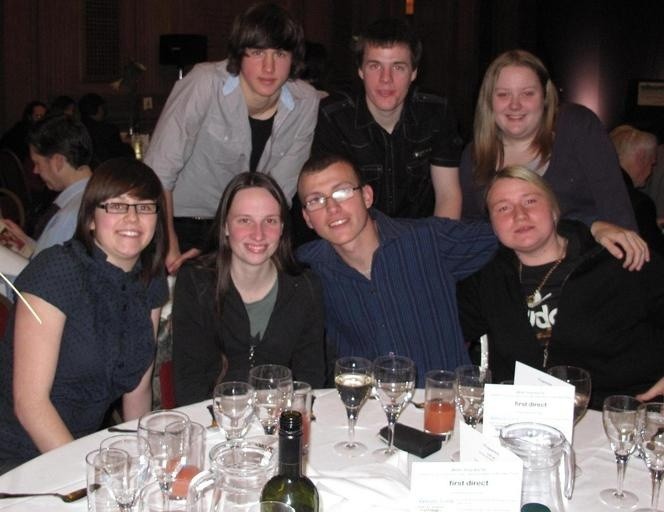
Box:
[0,479,102,505]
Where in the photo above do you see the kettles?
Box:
[500,424,577,508]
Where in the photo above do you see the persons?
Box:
[288,14,463,241]
[295,152,651,389]
[3,112,93,300]
[172,170,325,405]
[456,164,664,411]
[3,93,136,241]
[459,49,638,232]
[143,0,322,274]
[102,62,148,94]
[0,156,171,476]
[608,125,660,251]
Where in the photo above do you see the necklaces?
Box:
[519,237,567,302]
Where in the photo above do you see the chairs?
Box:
[1,289,13,341]
[0,144,42,237]
[0,188,26,229]
[467,330,491,383]
[151,275,178,412]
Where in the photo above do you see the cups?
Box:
[133,141,143,160]
[425,370,458,443]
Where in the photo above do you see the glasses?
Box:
[301,185,362,213]
[96,203,159,215]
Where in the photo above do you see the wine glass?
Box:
[334,358,373,457]
[599,395,645,508]
[635,400,663,512]
[374,358,417,461]
[85,364,315,510]
[458,364,492,431]
[550,368,593,428]
